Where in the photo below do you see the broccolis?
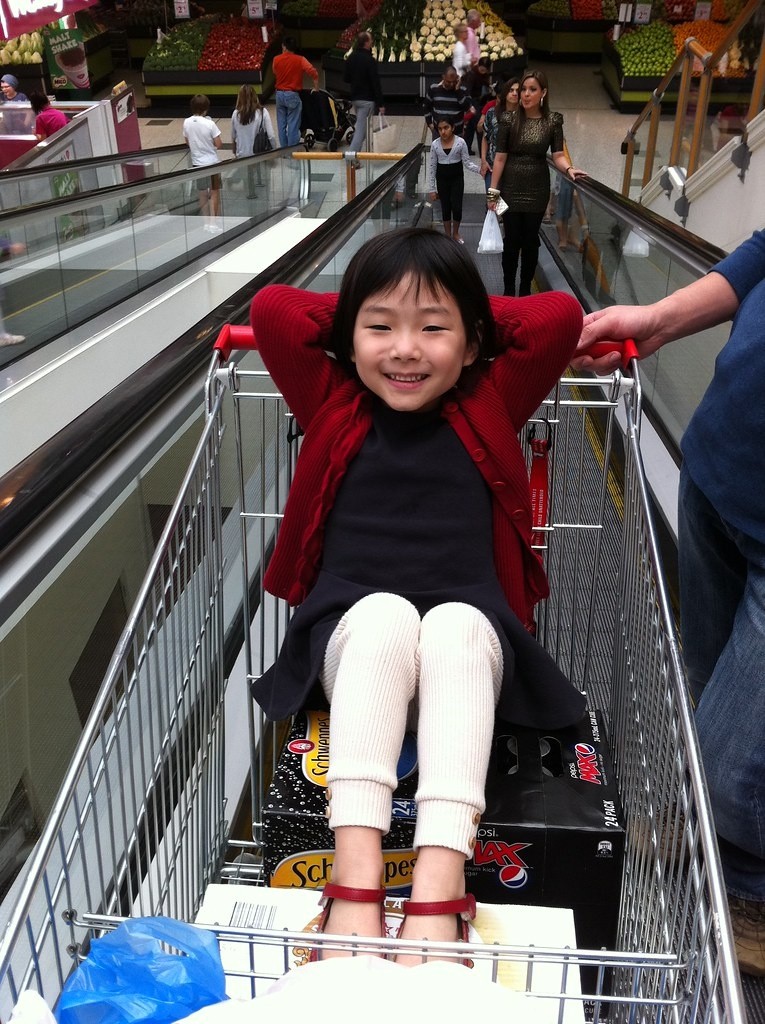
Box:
[475,25,524,61]
[409,0,467,62]
[143,17,225,71]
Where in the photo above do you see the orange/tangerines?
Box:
[673,18,747,78]
[570,0,604,21]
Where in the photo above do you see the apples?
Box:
[614,20,676,77]
[280,0,378,17]
[527,0,570,17]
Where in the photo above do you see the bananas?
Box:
[463,0,515,36]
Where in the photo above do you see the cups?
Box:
[55,43,90,89]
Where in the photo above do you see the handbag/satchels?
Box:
[253,128,274,166]
[371,112,397,153]
[476,208,504,254]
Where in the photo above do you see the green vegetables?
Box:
[344,0,427,63]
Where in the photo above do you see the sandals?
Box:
[391,893,476,967]
[310,882,388,963]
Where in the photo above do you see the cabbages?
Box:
[0,33,44,66]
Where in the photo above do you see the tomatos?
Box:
[198,17,279,71]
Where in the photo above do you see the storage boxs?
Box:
[260,708,628,1016]
[190,881,585,1024]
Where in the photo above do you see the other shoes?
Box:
[456,237,464,244]
[0,333,25,348]
[355,163,364,169]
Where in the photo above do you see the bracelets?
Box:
[565,167,574,173]
[486,193,500,202]
[488,188,500,194]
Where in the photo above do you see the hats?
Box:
[1,74,19,89]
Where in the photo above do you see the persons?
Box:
[0,74,34,135]
[230,83,278,229]
[0,239,26,347]
[371,176,405,234]
[569,221,764,858]
[272,37,319,158]
[345,32,386,169]
[544,167,586,253]
[251,225,583,965]
[422,9,588,299]
[31,91,71,141]
[225,167,242,192]
[182,93,223,216]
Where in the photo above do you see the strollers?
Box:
[296,87,357,153]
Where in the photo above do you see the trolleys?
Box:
[0,320,749,1024]
